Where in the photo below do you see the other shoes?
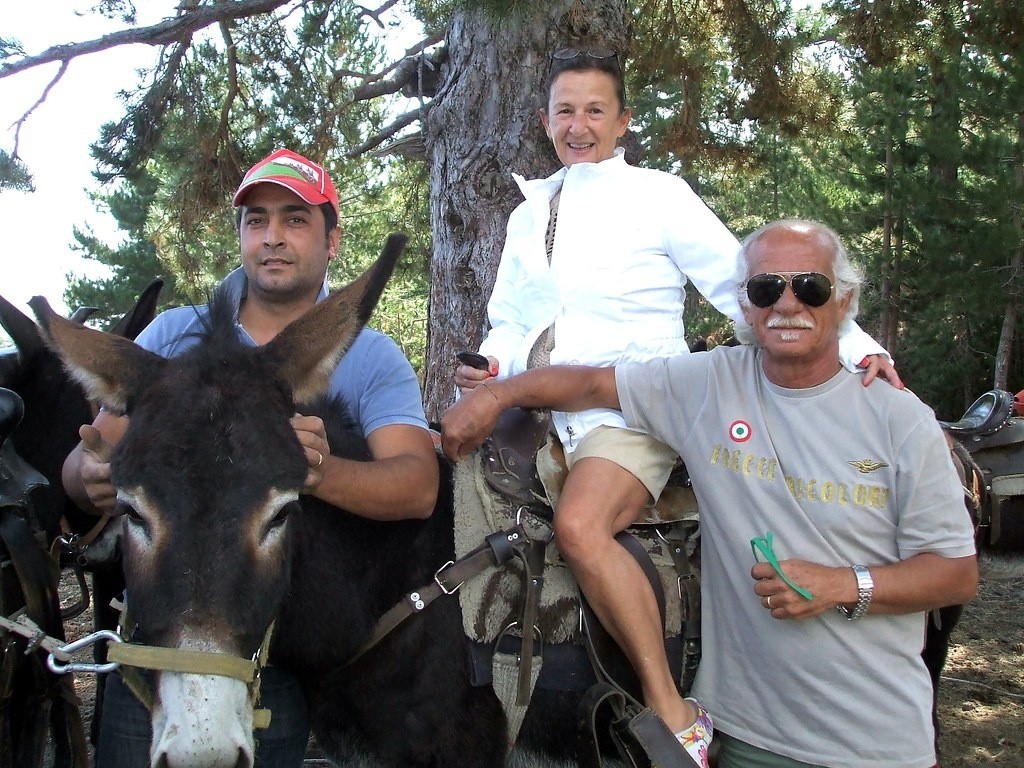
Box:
[674,694,714,768]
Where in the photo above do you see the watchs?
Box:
[835,563,874,622]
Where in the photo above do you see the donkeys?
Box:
[0,234,988,768]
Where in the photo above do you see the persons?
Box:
[455,43,903,768]
[63,148,440,768]
[438,219,978,768]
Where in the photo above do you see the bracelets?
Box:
[482,382,504,416]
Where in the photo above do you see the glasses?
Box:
[744,272,836,308]
[549,48,626,100]
[750,531,812,601]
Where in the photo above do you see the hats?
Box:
[233,148,339,225]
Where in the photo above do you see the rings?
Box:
[767,596,776,610]
[313,452,323,470]
